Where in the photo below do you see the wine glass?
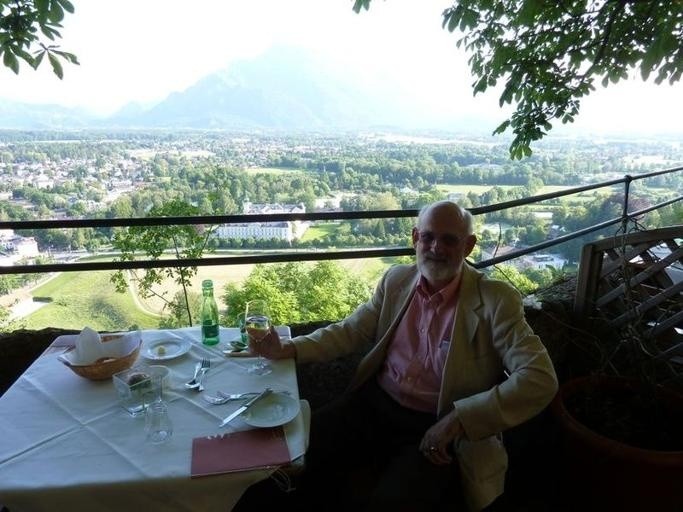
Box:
[244,299,273,377]
[143,365,172,446]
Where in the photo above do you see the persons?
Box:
[232,199,560,511]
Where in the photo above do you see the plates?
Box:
[239,393,300,428]
[140,337,192,362]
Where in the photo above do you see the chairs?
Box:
[443,303,556,512]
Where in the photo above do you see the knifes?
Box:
[217,388,272,429]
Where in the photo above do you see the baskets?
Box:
[62,333,143,381]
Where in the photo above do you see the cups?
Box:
[109,363,165,419]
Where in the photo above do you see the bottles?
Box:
[198,279,220,346]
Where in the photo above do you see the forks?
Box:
[198,356,211,392]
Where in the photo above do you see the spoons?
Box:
[203,388,288,405]
[184,360,201,389]
[229,340,249,351]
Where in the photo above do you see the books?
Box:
[188,425,293,480]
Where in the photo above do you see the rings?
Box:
[424,451,429,457]
[430,446,438,455]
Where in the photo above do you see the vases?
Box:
[552,370,683,512]
